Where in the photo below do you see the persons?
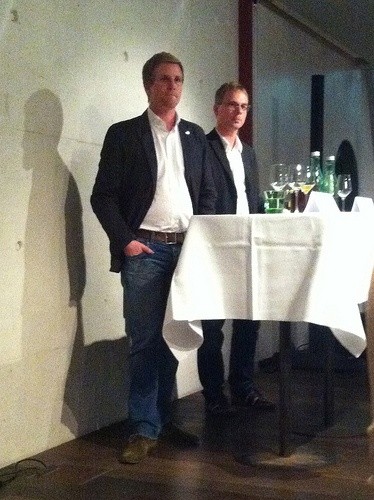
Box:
[90,52,217,463]
[197,81,276,418]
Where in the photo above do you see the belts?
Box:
[137,230,186,245]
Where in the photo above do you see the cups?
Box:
[264,191,285,212]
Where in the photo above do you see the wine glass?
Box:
[268,163,289,215]
[287,163,307,214]
[335,174,352,212]
[299,165,316,214]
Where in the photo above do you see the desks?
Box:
[162,212,374,459]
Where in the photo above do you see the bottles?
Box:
[307,151,323,192]
[321,155,335,193]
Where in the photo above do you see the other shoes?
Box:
[232,391,276,410]
[161,421,200,446]
[208,403,245,417]
[120,436,159,463]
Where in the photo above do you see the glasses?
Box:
[227,102,251,111]
